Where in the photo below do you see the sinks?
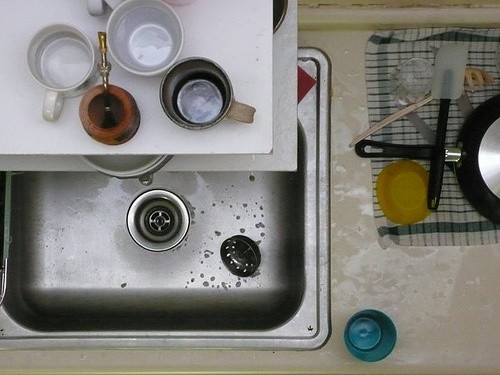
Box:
[0,46,331,352]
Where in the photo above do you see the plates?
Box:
[376,162,432,227]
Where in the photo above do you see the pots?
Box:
[355,91,500,228]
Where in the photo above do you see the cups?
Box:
[342,310,399,365]
[24,23,98,123]
[105,0,185,76]
[158,54,259,131]
[88,0,104,16]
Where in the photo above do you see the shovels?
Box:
[429,43,468,209]
